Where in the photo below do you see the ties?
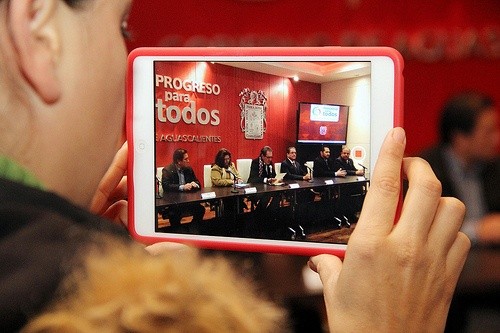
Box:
[293,163,295,167]
[267,164,270,176]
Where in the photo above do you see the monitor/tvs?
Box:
[295,102,349,145]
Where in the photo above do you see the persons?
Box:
[280,146,312,207]
[210,148,248,235]
[158,148,205,234]
[22,229,288,333]
[312,146,347,200]
[0,0,470,333]
[247,145,284,210]
[335,148,365,175]
[404,90,500,247]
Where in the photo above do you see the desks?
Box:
[156,175,370,230]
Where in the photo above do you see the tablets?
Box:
[126,46,406,262]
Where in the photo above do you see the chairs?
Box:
[305,161,314,177]
[203,164,216,211]
[157,166,164,192]
[274,162,285,207]
[236,157,252,182]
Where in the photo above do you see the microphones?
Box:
[359,164,367,169]
[226,170,237,178]
[304,163,312,171]
[269,165,273,173]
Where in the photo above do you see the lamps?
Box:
[283,74,301,83]
